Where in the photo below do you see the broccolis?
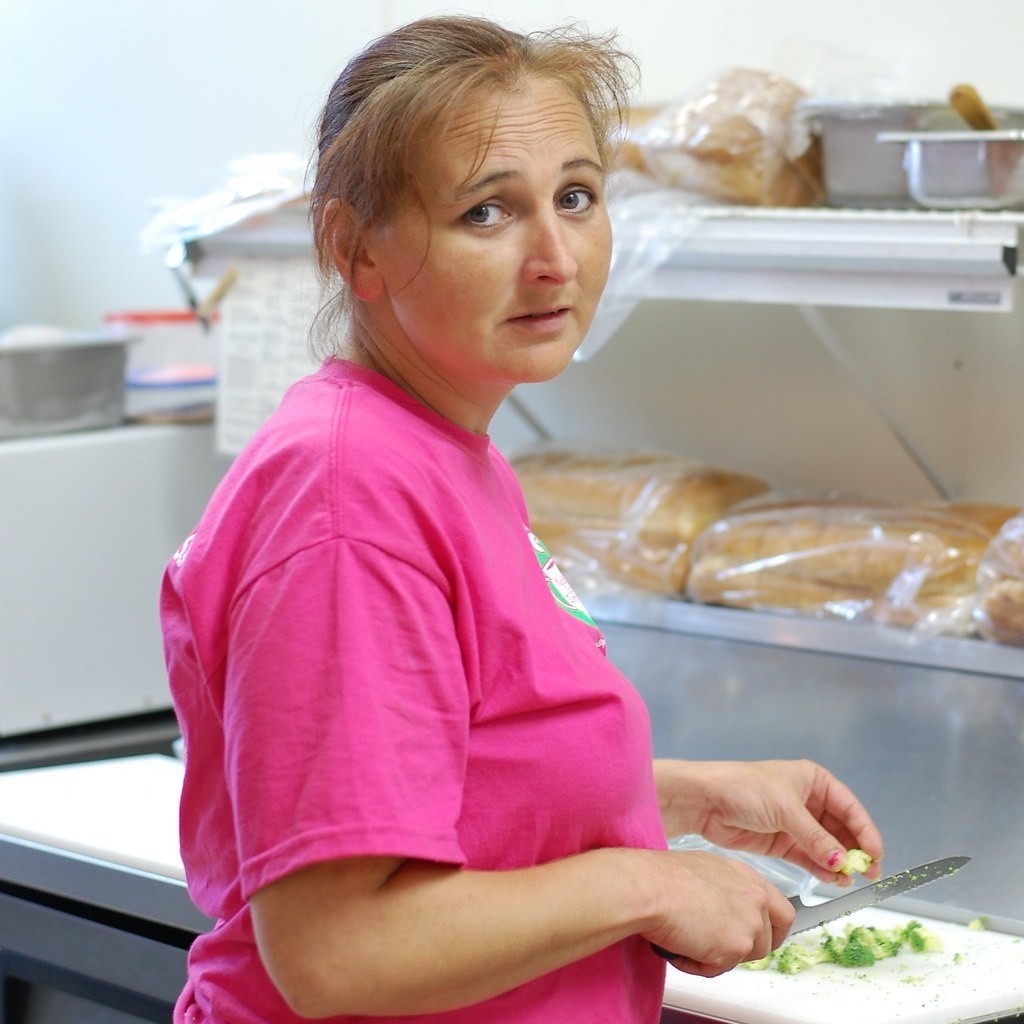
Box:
[740,850,985,974]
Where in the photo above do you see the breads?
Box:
[600,64,826,208]
[502,448,1024,653]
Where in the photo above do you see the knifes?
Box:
[655,855,970,961]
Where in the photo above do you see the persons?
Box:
[158,18,882,1024]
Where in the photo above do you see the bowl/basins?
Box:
[880,132,1024,212]
[795,99,1024,210]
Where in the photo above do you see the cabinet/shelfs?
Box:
[152,102,1024,680]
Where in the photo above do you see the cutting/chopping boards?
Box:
[662,894,1024,1024]
